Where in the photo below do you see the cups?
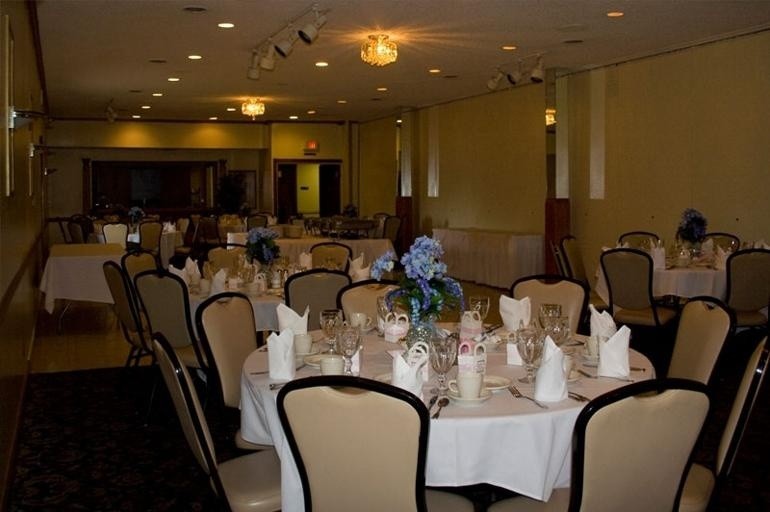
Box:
[448,373,483,398]
[294,334,312,354]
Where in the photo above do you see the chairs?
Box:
[133,269,209,388]
[100,260,157,384]
[3,215,769,328]
[192,294,276,448]
[153,336,282,511]
[676,337,770,512]
[276,377,479,510]
[490,378,711,512]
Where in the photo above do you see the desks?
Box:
[238,322,659,510]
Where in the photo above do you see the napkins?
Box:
[532,335,571,405]
[595,325,632,378]
[589,304,618,352]
[274,302,310,363]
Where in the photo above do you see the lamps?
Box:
[105,104,117,125]
[241,98,266,119]
[544,108,556,134]
[247,1,328,80]
[360,32,398,67]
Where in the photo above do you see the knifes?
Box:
[427,396,437,411]
[584,364,646,372]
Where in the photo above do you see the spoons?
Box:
[431,398,449,420]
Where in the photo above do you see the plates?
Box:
[304,354,342,369]
[375,374,393,385]
[446,391,491,404]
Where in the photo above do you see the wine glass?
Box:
[319,296,570,395]
[203,253,343,290]
[639,238,764,267]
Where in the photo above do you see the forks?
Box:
[507,386,549,410]
[578,369,634,382]
